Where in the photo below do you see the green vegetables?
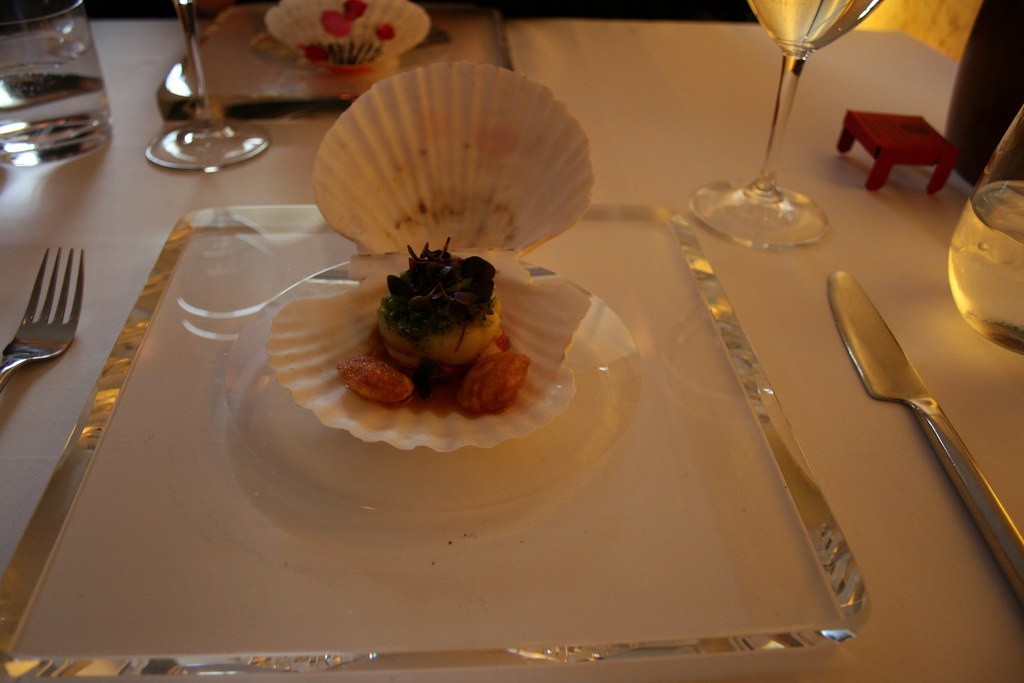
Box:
[379,236,499,344]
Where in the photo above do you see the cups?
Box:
[0,0,114,167]
[947,103,1024,355]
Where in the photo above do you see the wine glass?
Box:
[143,0,271,170]
[691,0,886,249]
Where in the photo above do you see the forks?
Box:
[0,245,87,392]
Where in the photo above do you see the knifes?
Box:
[825,268,1024,605]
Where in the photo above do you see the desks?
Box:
[0,19,1024,683]
